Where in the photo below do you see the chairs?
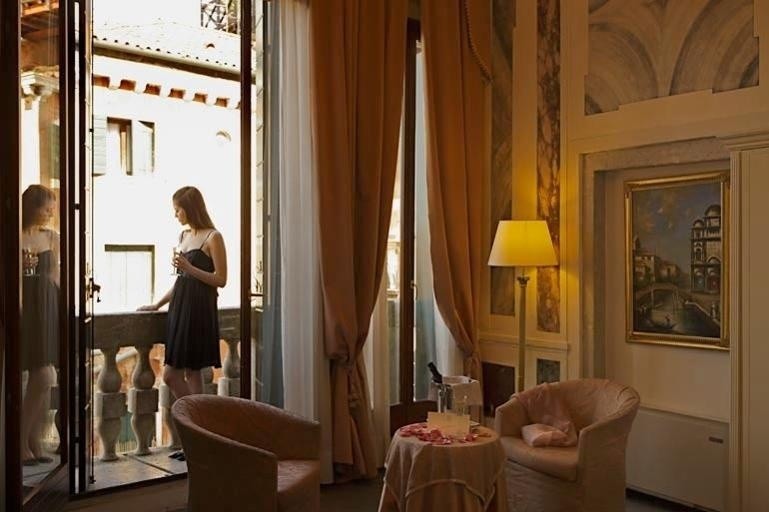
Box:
[491,376,643,512]
[169,392,325,512]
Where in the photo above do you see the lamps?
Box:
[484,217,559,402]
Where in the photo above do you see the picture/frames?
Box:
[620,168,733,354]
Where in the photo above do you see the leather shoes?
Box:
[22,457,53,466]
[168,451,186,461]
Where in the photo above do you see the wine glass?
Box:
[170,244,186,277]
[23,244,38,277]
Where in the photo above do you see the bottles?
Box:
[427,362,442,383]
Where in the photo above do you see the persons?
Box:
[20,185,64,465]
[134,186,227,460]
